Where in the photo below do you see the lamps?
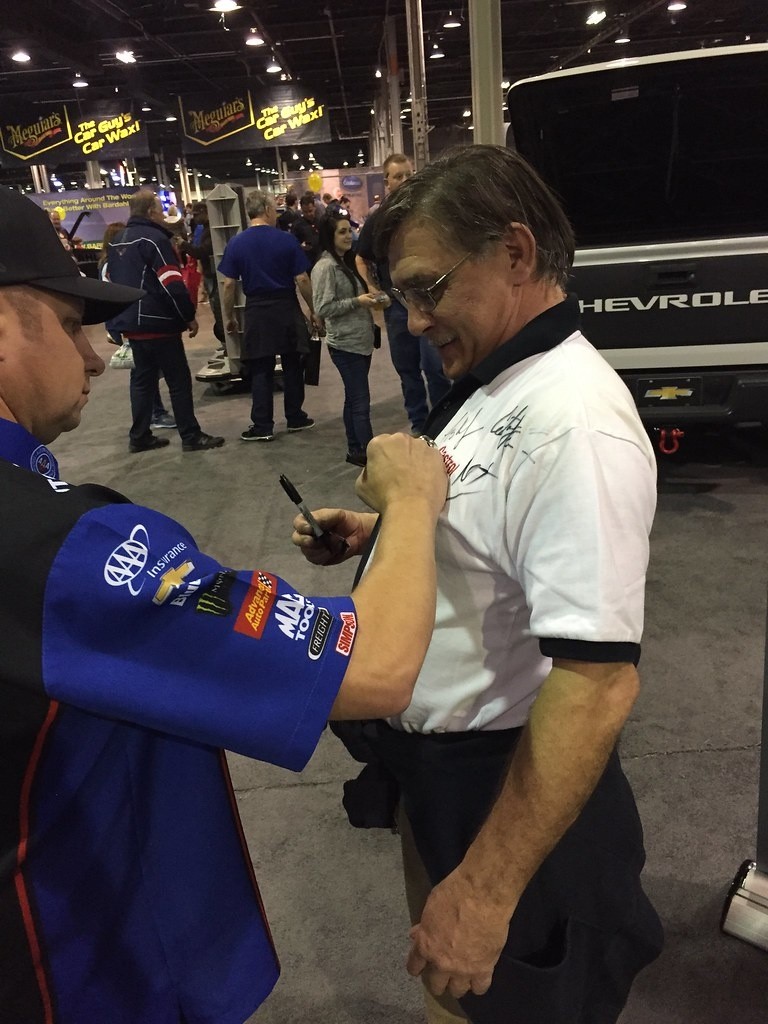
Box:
[246,27,283,72]
[443,10,462,28]
[71,72,89,88]
[429,44,445,58]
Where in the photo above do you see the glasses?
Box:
[390,237,489,314]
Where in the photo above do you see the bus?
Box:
[368,1,768,477]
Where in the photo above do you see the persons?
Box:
[0,153,451,1024]
[299,148,669,1024]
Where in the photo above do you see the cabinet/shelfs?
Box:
[195,184,287,393]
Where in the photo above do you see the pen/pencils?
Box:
[277,473,344,562]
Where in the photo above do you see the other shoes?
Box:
[345,450,369,468]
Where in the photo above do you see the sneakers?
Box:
[129,437,170,453]
[153,412,179,429]
[287,418,315,434]
[240,423,274,440]
[182,430,225,452]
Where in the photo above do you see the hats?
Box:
[0,185,147,325]
[186,203,208,214]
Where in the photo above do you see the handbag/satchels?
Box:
[292,324,321,386]
